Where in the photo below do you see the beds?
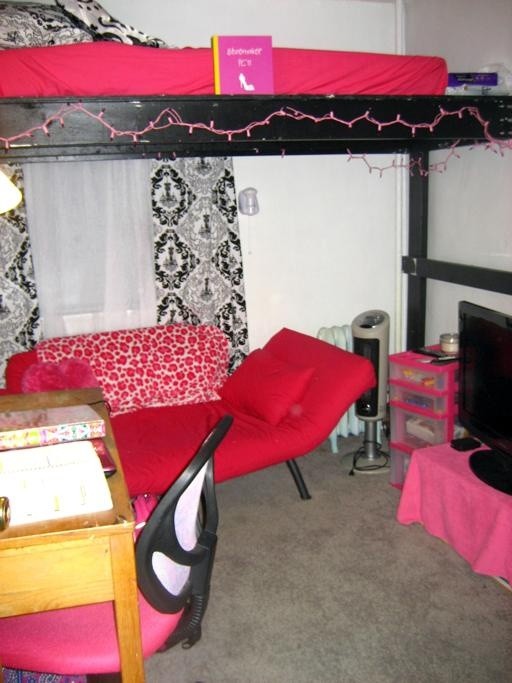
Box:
[110,322,378,498]
[2,42,512,354]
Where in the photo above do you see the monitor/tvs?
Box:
[457,299,512,500]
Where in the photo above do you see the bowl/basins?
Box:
[439,333,459,354]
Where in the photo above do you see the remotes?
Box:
[411,347,448,358]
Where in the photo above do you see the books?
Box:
[0,403,107,451]
[210,34,274,94]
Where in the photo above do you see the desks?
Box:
[409,434,512,589]
[1,386,148,682]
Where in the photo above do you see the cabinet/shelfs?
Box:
[387,344,460,494]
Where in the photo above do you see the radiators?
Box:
[315,323,390,455]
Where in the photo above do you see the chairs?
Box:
[0,413,235,682]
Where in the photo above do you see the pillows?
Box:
[21,357,101,394]
[216,345,315,424]
